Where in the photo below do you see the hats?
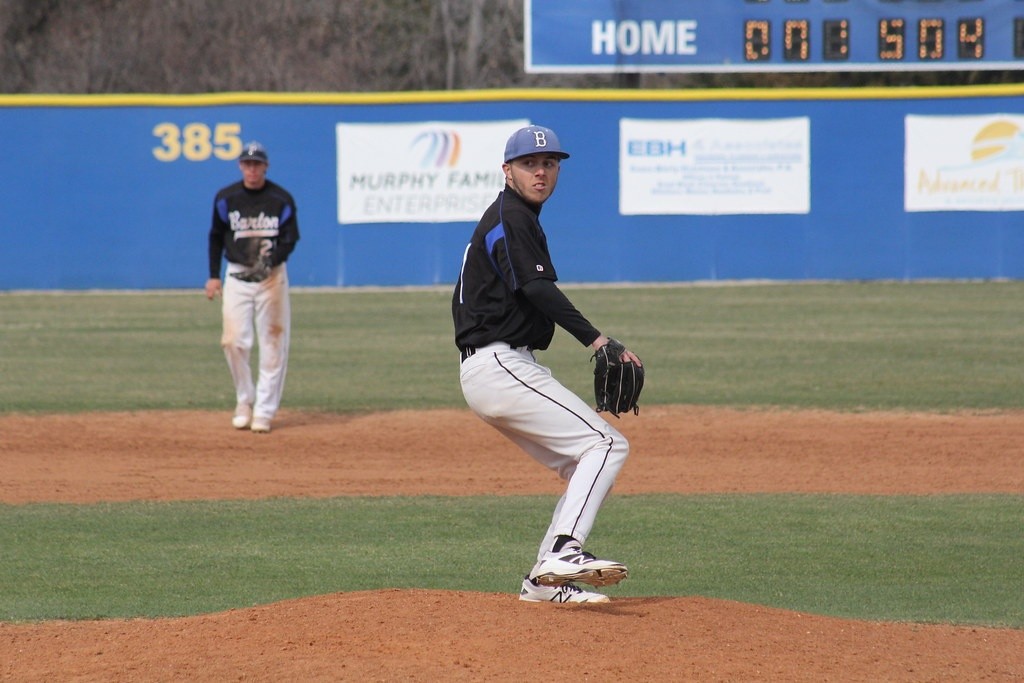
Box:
[504,124,570,163]
[240,141,268,164]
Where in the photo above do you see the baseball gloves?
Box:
[590,336,645,422]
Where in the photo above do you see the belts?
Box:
[462,343,517,364]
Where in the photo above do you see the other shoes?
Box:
[233,403,252,428]
[251,415,271,433]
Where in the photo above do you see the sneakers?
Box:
[519,575,611,603]
[535,549,628,588]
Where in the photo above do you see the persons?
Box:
[205,141,301,433]
[451,124,645,604]
